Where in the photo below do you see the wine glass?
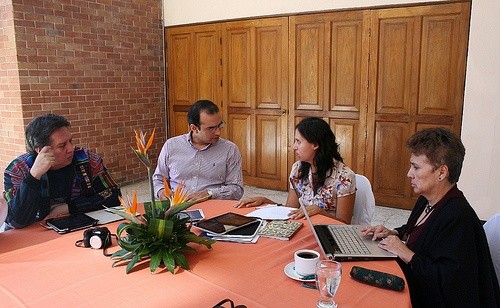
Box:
[315,260,342,308]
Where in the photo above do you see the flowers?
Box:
[102,129,216,275]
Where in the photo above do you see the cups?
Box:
[295,249,320,276]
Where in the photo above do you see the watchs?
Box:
[207,190,212,200]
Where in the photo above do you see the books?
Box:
[45,213,99,235]
[193,212,304,243]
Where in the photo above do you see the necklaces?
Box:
[404,200,438,237]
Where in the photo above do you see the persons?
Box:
[233,116,357,225]
[0,113,123,232]
[360,127,500,308]
[152,99,245,203]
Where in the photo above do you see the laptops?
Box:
[289,177,401,262]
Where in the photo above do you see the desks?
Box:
[0,200,412,308]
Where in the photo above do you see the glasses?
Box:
[194,119,227,133]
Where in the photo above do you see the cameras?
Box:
[83,226,113,248]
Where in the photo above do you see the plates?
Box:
[284,262,316,281]
